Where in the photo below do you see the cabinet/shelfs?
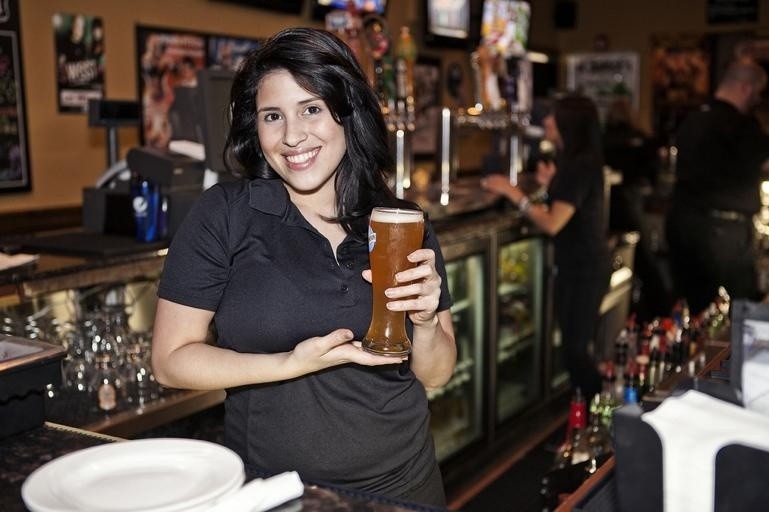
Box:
[419,214,556,462]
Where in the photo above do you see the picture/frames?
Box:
[134,20,267,150]
[410,55,445,158]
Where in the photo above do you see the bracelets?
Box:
[518,196,531,213]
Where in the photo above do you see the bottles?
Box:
[361,205,426,358]
[365,54,417,129]
[533,285,733,512]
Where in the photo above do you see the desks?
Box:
[1,419,454,510]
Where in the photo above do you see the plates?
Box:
[19,434,249,512]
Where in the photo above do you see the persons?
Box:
[148,23,463,511]
[479,94,615,453]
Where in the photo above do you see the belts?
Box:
[712,209,750,225]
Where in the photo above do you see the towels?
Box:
[196,466,306,510]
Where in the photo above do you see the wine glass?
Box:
[1,299,160,408]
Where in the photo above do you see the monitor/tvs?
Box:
[421,0,484,52]
[195,67,246,173]
